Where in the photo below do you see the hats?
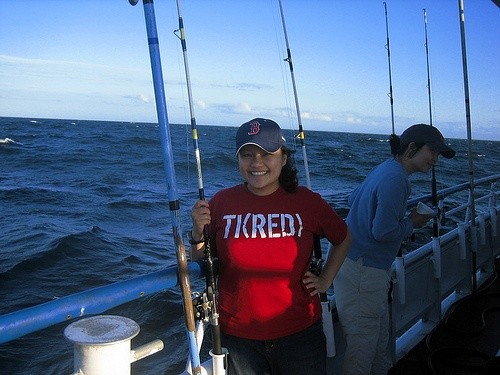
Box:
[234,118,284,158]
[400,124,456,159]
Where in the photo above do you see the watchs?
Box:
[187,230,208,245]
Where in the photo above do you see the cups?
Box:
[417,201,435,214]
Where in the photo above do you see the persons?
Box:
[326,124,455,375]
[186,117,353,375]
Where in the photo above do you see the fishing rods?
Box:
[423,9,440,238]
[278,2,328,301]
[384,2,404,259]
[459,1,479,295]
[175,1,224,355]
[130,1,205,375]
